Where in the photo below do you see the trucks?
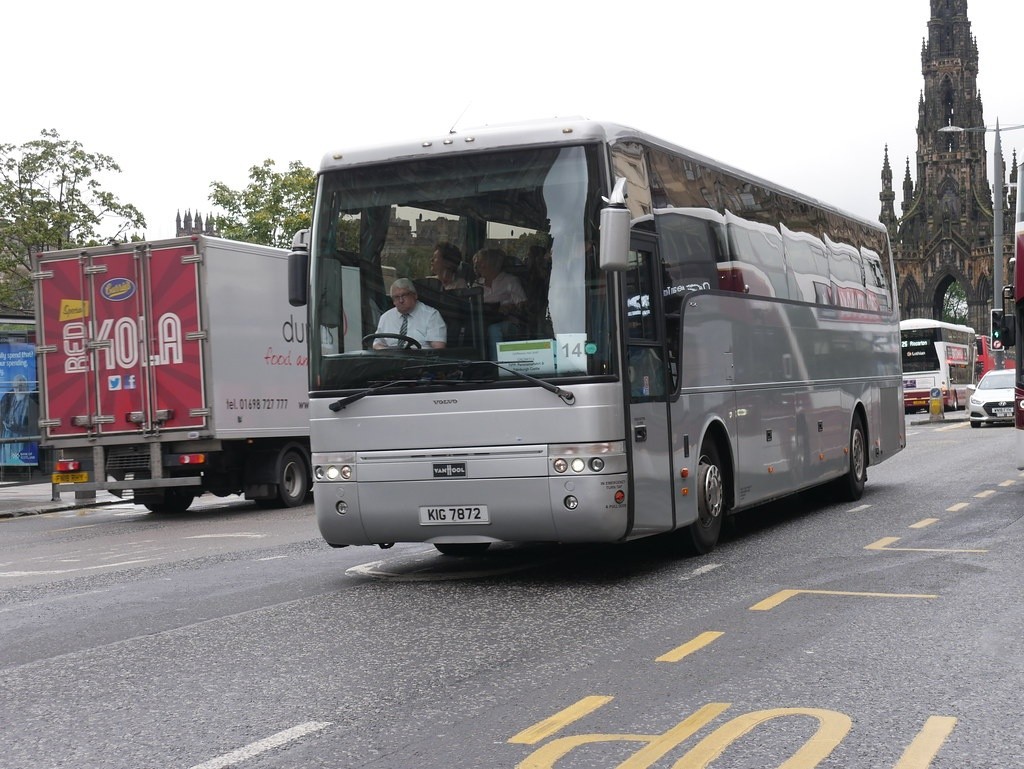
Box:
[31,233,399,518]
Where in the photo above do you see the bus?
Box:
[286,119,910,555]
[899,317,979,413]
[1000,231,1024,430]
[974,333,1016,383]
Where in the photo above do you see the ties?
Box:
[398,314,407,347]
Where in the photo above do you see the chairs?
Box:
[460,245,547,291]
[408,273,450,334]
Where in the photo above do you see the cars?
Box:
[969,367,1018,429]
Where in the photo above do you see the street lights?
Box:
[936,115,1024,369]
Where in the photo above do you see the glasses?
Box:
[392,291,413,302]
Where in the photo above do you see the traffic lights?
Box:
[991,309,1003,352]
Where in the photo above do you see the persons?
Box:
[429,242,469,289]
[373,277,447,349]
[519,246,550,340]
[0,375,40,464]
[470,246,526,361]
